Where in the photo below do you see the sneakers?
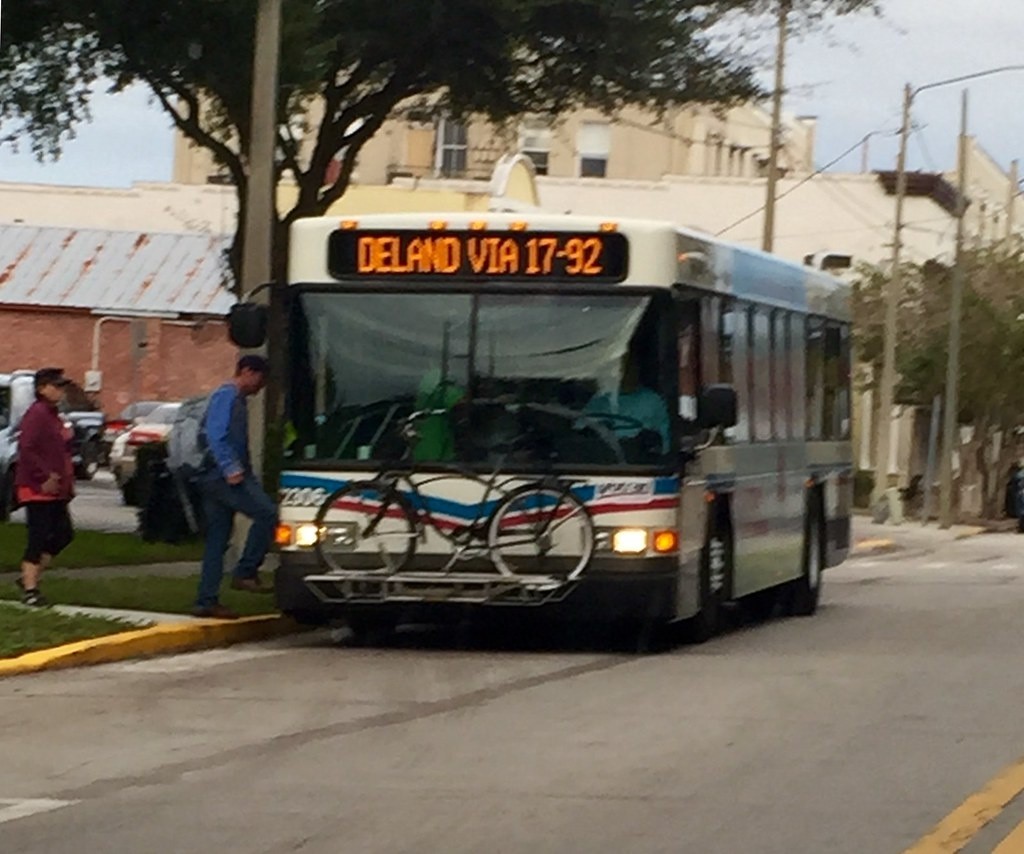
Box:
[191,604,238,619]
[229,576,274,594]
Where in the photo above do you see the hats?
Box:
[33,368,73,386]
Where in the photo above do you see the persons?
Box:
[572,344,670,464]
[15,368,78,608]
[407,338,466,463]
[192,354,279,619]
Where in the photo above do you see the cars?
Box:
[0,367,185,524]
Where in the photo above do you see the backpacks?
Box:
[164,380,243,476]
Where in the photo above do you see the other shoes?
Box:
[15,577,47,606]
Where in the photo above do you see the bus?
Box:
[223,199,864,647]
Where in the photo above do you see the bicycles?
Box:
[313,407,599,598]
